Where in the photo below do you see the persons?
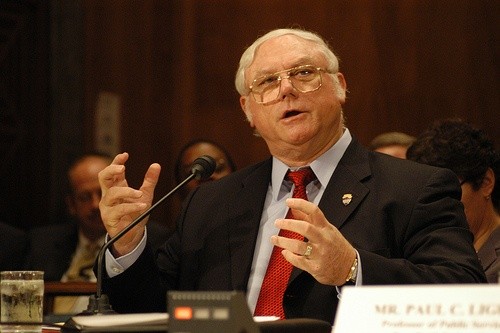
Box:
[406,121,500,283]
[372,132,416,159]
[97,27,486,327]
[176,141,235,197]
[25,154,172,316]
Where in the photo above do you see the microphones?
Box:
[60,154,216,333]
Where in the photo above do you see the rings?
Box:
[303,242,312,256]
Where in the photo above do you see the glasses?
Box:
[246,65,330,104]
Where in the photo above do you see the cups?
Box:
[0,323,43,333]
[0,270,46,323]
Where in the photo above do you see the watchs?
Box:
[336,259,358,292]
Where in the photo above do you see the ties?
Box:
[53,244,99,325]
[254,167,317,320]
